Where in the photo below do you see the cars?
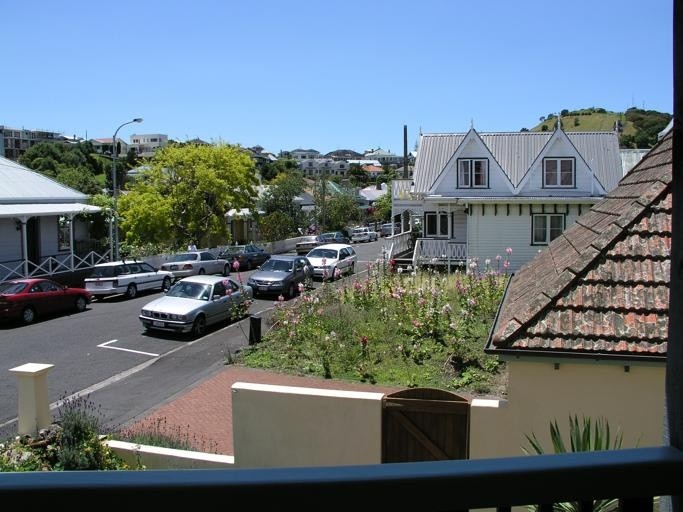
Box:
[246,255,313,299]
[0,278,90,326]
[296,218,422,254]
[138,274,253,336]
[216,245,270,270]
[160,251,231,280]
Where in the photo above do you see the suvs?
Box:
[84,261,174,298]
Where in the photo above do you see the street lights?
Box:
[112,117,143,260]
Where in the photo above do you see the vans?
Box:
[302,244,357,281]
